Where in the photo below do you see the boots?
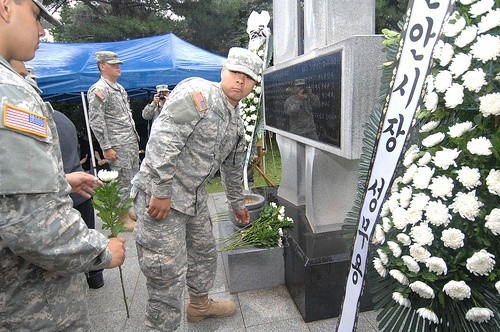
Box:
[117,207,138,231]
[187,293,236,322]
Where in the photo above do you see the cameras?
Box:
[157,91,166,100]
[301,87,309,94]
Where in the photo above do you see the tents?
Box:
[22,32,227,138]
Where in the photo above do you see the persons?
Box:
[78,138,148,171]
[0,0,126,332]
[131,46,264,332]
[87,51,140,232]
[142,85,172,122]
[284,79,320,141]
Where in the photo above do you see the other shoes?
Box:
[88,278,104,289]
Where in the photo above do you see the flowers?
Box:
[340,0,500,332]
[93,169,142,320]
[239,8,274,148]
[216,199,295,256]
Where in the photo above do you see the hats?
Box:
[33,0,62,27]
[294,78,305,86]
[95,52,123,64]
[226,47,264,82]
[26,65,37,78]
[156,85,170,92]
